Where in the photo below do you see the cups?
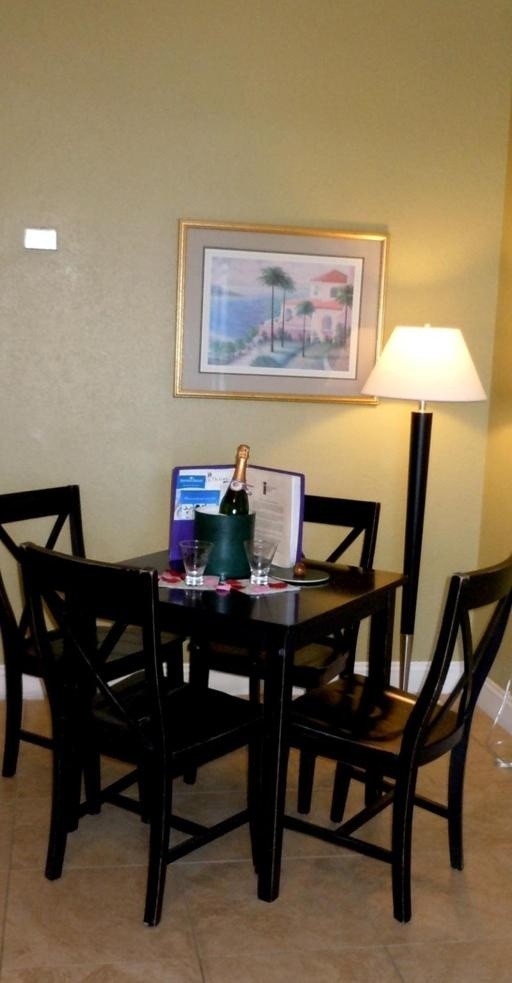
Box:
[243,538,278,587]
[178,539,214,586]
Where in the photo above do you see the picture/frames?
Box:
[172,219,391,407]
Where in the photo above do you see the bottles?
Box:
[217,444,252,517]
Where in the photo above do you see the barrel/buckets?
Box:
[194,503,257,578]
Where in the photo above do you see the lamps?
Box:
[362,321,486,693]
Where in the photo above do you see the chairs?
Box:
[14,540,262,926]
[4,485,162,832]
[271,555,512,930]
[188,496,380,819]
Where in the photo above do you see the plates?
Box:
[269,568,331,584]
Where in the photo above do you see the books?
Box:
[169,462,305,566]
